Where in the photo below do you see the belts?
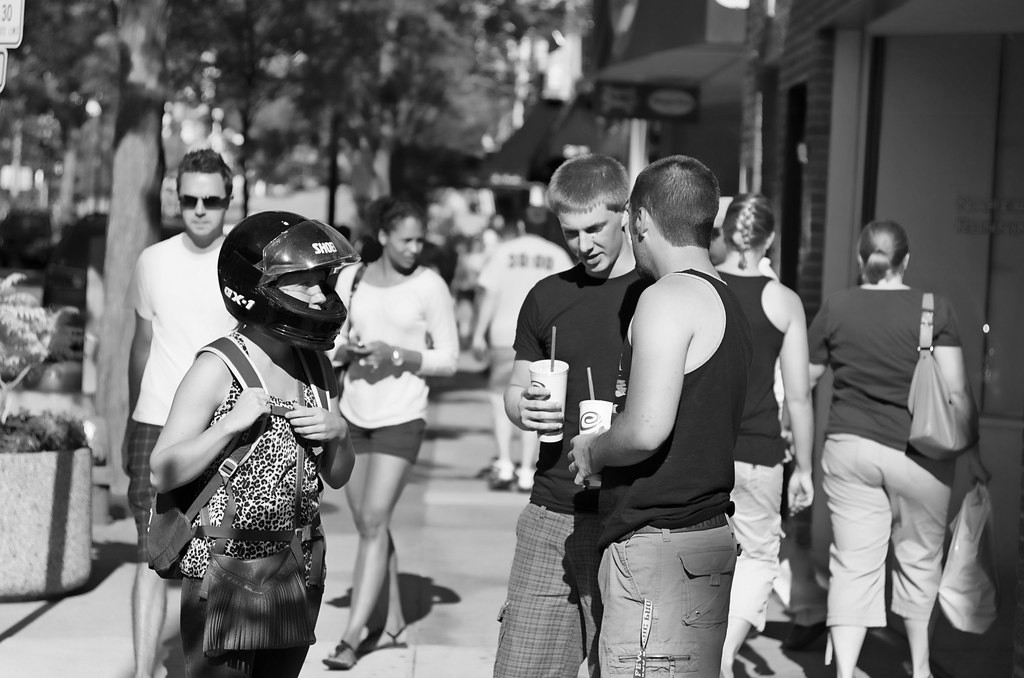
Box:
[634,513,728,534]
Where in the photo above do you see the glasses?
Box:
[178,195,228,207]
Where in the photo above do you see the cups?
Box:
[577,399,614,487]
[528,358,569,443]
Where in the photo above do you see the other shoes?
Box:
[489,457,515,489]
[514,463,534,493]
[783,621,827,650]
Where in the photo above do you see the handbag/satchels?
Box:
[908,292,983,461]
[203,547,317,659]
[938,482,1001,635]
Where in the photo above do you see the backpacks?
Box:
[146,335,332,579]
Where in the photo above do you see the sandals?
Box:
[322,633,371,670]
[358,625,408,656]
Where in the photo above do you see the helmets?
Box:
[217,211,362,351]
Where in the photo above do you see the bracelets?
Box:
[391,346,404,367]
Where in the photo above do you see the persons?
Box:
[470,205,575,491]
[148,212,355,678]
[123,149,242,678]
[325,200,460,666]
[712,194,814,678]
[493,155,657,678]
[568,155,744,678]
[806,219,990,678]
